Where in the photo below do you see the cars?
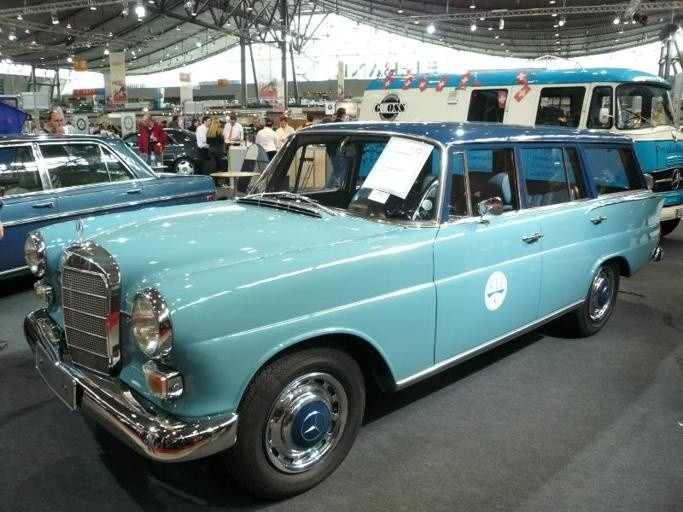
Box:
[23,122,665,498]
[123,128,211,174]
[0,132,217,282]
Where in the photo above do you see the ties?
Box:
[228,126,233,141]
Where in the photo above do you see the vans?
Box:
[358,67,683,236]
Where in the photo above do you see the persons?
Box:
[140,108,346,189]
[40,111,76,134]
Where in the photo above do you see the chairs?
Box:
[462,170,513,211]
[3,169,39,195]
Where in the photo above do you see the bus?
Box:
[125,97,154,110]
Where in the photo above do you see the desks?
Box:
[209,172,260,197]
